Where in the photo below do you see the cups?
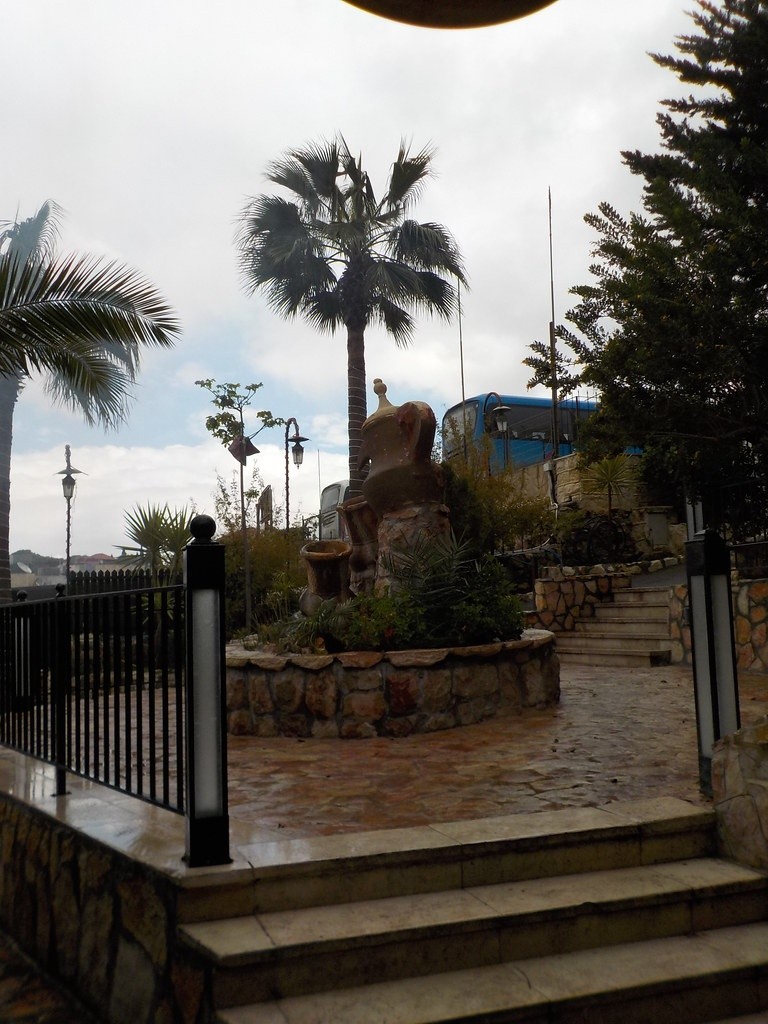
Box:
[299,539,353,596]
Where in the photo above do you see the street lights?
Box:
[54,444,88,595]
[483,392,511,478]
[284,416,310,578]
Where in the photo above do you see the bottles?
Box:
[336,494,379,573]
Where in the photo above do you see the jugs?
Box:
[356,378,445,518]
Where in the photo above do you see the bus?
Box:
[442,395,644,480]
